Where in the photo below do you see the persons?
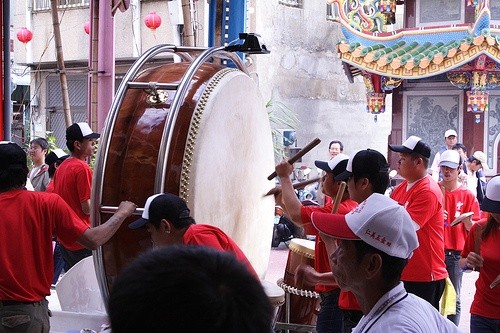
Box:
[0,141,139,333]
[52,122,102,265]
[129,193,260,280]
[28,138,50,192]
[274,130,500,333]
[109,246,274,333]
[44,149,70,290]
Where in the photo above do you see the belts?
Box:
[444,251,460,255]
[3,300,33,306]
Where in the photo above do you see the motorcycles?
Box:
[271,165,316,249]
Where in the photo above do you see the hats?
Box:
[439,150,462,169]
[0,141,27,169]
[335,149,389,181]
[45,149,70,164]
[311,193,419,260]
[66,122,100,142]
[389,136,431,157]
[482,175,500,214]
[128,192,190,229]
[445,129,457,140]
[315,153,352,174]
[473,151,490,171]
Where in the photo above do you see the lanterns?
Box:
[144,12,162,31]
[84,21,90,34]
[17,27,32,46]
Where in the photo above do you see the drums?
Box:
[88,42,286,328]
[282,237,319,330]
